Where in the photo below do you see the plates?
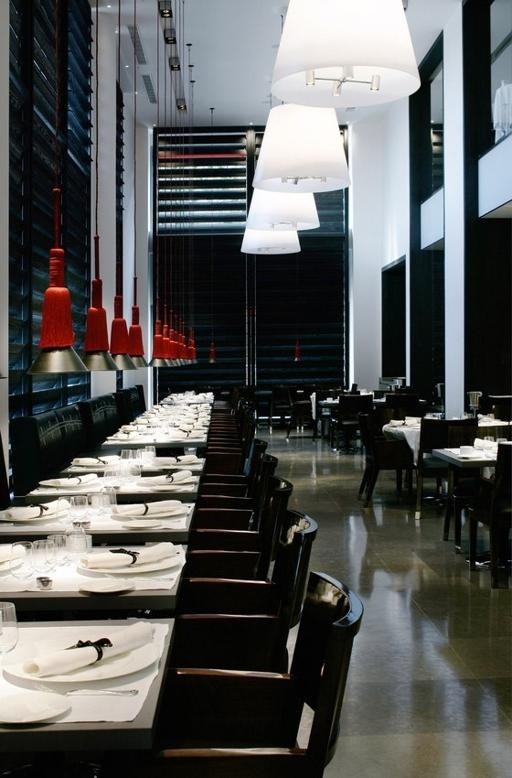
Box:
[76,544,181,575]
[139,476,194,485]
[456,454,472,458]
[107,388,213,447]
[155,459,203,466]
[0,689,72,725]
[2,626,165,683]
[1,509,69,522]
[109,502,189,519]
[0,555,24,572]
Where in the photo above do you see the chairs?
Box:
[1,379,512,776]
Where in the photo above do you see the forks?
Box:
[40,685,140,696]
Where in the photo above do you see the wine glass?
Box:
[390,412,442,426]
[12,444,160,593]
[0,601,20,665]
[472,434,510,460]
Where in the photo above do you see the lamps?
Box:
[245,181,322,232]
[266,3,425,110]
[252,103,351,196]
[239,228,302,255]
[293,257,302,362]
[27,1,216,377]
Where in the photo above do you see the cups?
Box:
[326,397,333,403]
[460,446,472,454]
